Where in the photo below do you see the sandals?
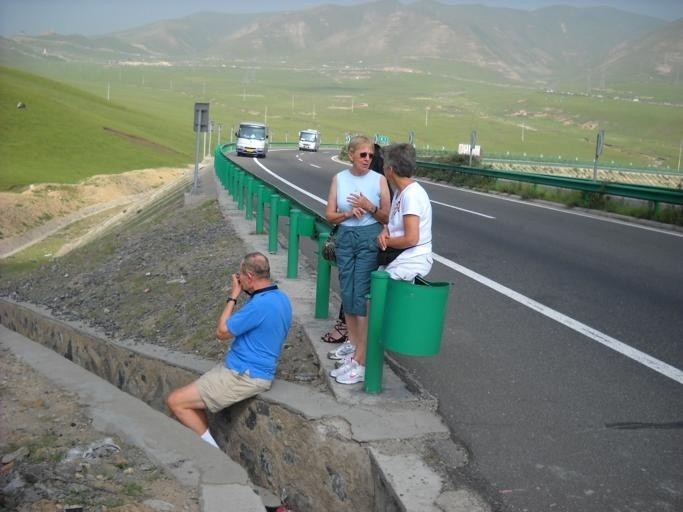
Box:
[321,320,349,343]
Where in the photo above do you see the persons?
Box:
[327,142,433,385]
[165,251,293,448]
[326,135,390,369]
[320,142,391,343]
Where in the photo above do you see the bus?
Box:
[234,122,269,157]
[297,129,320,152]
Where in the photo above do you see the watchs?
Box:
[225,296,237,305]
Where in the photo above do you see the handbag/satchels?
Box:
[322,236,337,261]
[376,245,402,266]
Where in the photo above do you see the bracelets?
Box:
[370,206,377,214]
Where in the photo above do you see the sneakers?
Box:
[328,339,365,384]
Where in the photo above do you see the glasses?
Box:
[360,151,374,159]
